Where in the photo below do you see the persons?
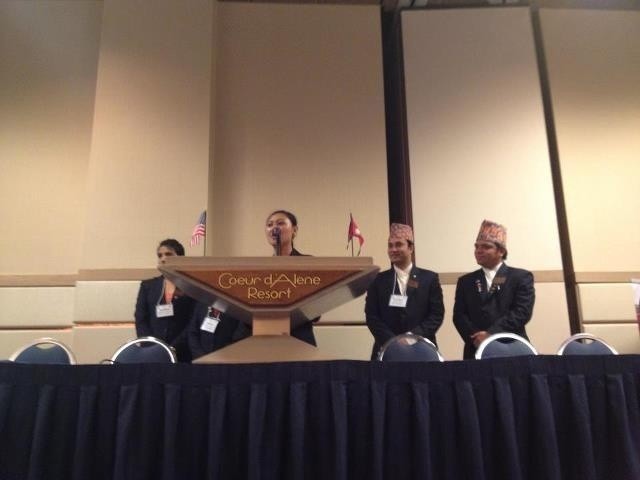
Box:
[451,219,537,361]
[186,297,252,359]
[266,210,320,348]
[363,221,445,361]
[133,238,194,365]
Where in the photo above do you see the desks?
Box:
[1,353,640,480]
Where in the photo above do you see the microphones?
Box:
[271,227,281,257]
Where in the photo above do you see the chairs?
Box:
[10,338,77,366]
[110,336,179,365]
[376,333,617,361]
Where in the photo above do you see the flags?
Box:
[344,213,365,257]
[188,209,208,248]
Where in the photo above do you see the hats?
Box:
[476,219,508,260]
[389,222,415,247]
